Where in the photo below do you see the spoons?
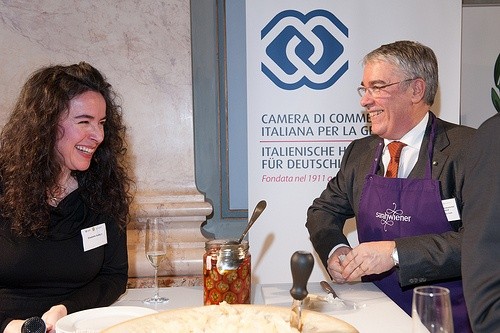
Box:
[216,199,267,276]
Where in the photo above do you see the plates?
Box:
[55,305,158,333]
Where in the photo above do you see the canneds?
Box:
[202,238,252,305]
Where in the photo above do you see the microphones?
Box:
[21,316,46,333]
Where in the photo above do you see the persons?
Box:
[0,61,137,333]
[306,41,478,333]
[461,113,500,333]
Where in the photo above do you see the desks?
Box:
[109,282,430,333]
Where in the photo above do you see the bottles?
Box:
[202,238,251,306]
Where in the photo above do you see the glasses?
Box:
[357,76,423,99]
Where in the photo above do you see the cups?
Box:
[411,285,454,333]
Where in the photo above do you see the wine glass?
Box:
[145,217,170,304]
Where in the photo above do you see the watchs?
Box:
[391,241,400,266]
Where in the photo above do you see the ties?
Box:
[386,141,407,178]
